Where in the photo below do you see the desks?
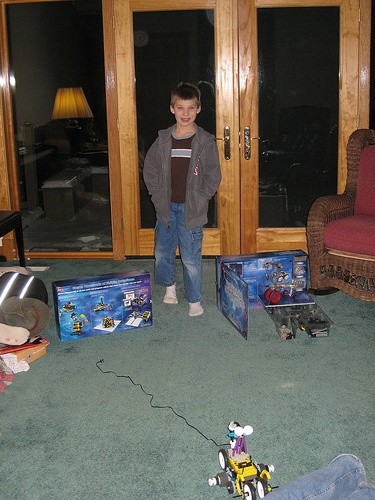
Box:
[0,211,26,267]
[39,166,109,221]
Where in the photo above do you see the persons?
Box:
[143,80,221,318]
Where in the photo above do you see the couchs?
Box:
[307,128,375,303]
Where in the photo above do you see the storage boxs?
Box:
[53,269,153,341]
[210,252,311,338]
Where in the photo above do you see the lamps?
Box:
[51,87,95,153]
